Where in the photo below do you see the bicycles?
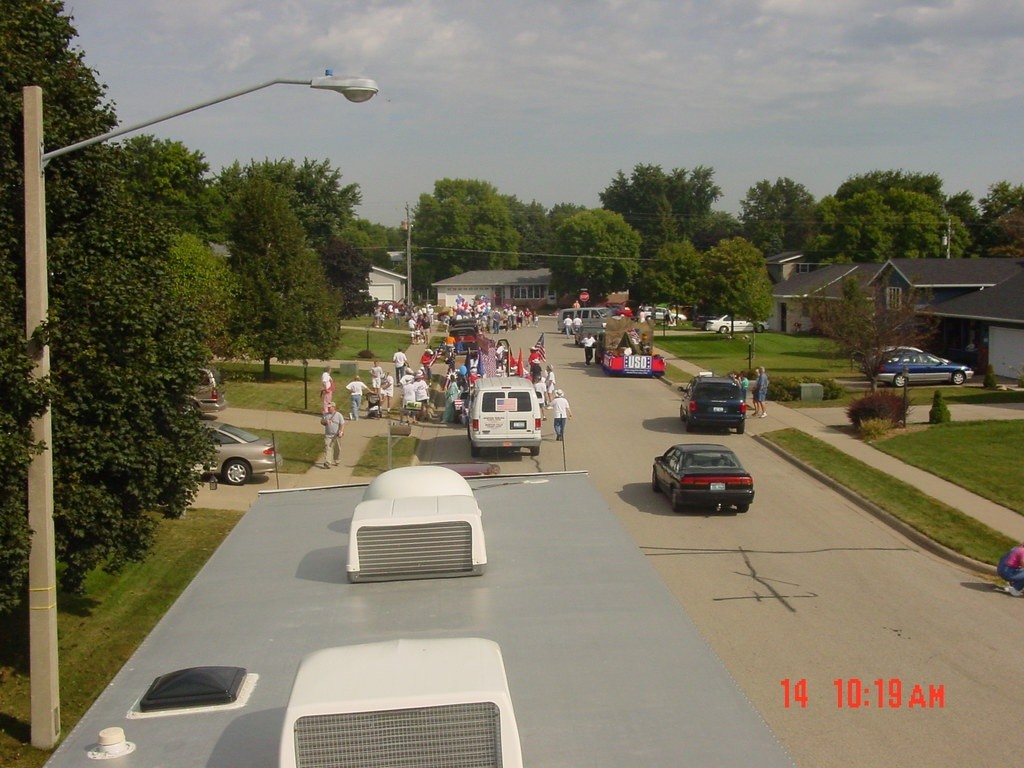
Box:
[447,348,452,361]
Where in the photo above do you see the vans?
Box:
[556,307,616,332]
[459,378,543,456]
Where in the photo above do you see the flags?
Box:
[535,334,546,363]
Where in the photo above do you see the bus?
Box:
[42,464,797,768]
[449,311,481,355]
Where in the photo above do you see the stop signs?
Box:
[580,292,589,302]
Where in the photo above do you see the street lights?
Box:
[25,64,376,750]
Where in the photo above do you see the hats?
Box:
[532,359,540,364]
[547,364,553,371]
[554,389,564,397]
[325,401,336,408]
[425,348,433,354]
[528,347,537,350]
[355,375,360,379]
[404,367,425,385]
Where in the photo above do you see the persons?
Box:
[546,389,572,441]
[320,346,556,426]
[730,366,768,417]
[581,333,597,367]
[373,299,539,345]
[573,300,581,309]
[495,342,505,370]
[320,401,345,469]
[444,333,456,362]
[564,314,582,339]
[997,543,1024,597]
[651,304,679,326]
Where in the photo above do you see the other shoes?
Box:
[589,365,592,367]
[354,418,358,421]
[556,437,563,441]
[543,418,547,421]
[388,408,390,412]
[752,412,760,416]
[584,363,587,367]
[547,406,553,410]
[428,382,432,387]
[324,462,331,469]
[349,412,353,420]
[761,413,767,418]
[335,463,341,467]
[1004,585,1023,596]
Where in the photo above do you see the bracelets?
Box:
[338,431,343,434]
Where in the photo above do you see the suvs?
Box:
[194,365,229,414]
[642,306,687,322]
[594,329,605,364]
[677,373,745,434]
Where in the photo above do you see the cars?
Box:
[861,348,974,385]
[695,311,770,335]
[376,299,402,315]
[652,447,754,513]
[600,302,634,319]
[195,423,282,483]
